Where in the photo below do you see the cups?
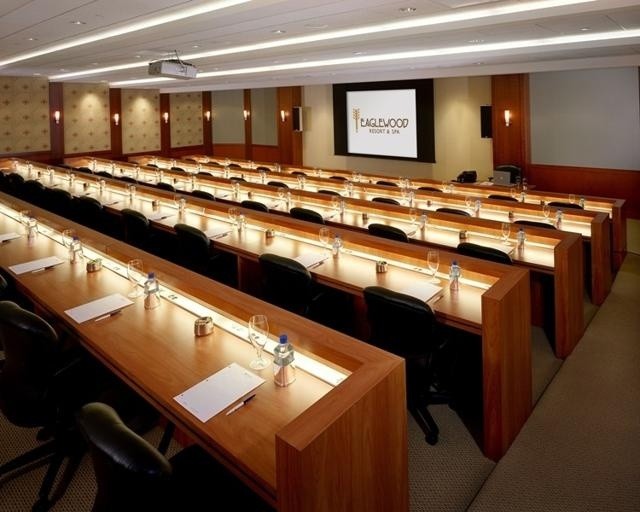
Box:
[62,229,74,250]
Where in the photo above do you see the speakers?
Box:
[479,104,493,139]
[292,106,303,132]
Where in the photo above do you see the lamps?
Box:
[114,113,119,126]
[280,110,287,123]
[164,112,169,124]
[504,109,512,128]
[206,111,211,122]
[54,111,60,125]
[244,110,248,121]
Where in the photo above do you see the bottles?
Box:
[144,271,161,309]
[86,258,101,273]
[517,227,524,251]
[70,235,83,264]
[194,316,214,336]
[375,260,387,273]
[333,235,342,258]
[273,335,297,388]
[451,261,462,292]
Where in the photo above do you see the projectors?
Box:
[148,59,196,80]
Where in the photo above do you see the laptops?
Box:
[493,170,517,187]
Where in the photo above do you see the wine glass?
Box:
[248,315,271,370]
[427,251,441,284]
[127,260,143,300]
[318,228,330,258]
[12,156,585,245]
